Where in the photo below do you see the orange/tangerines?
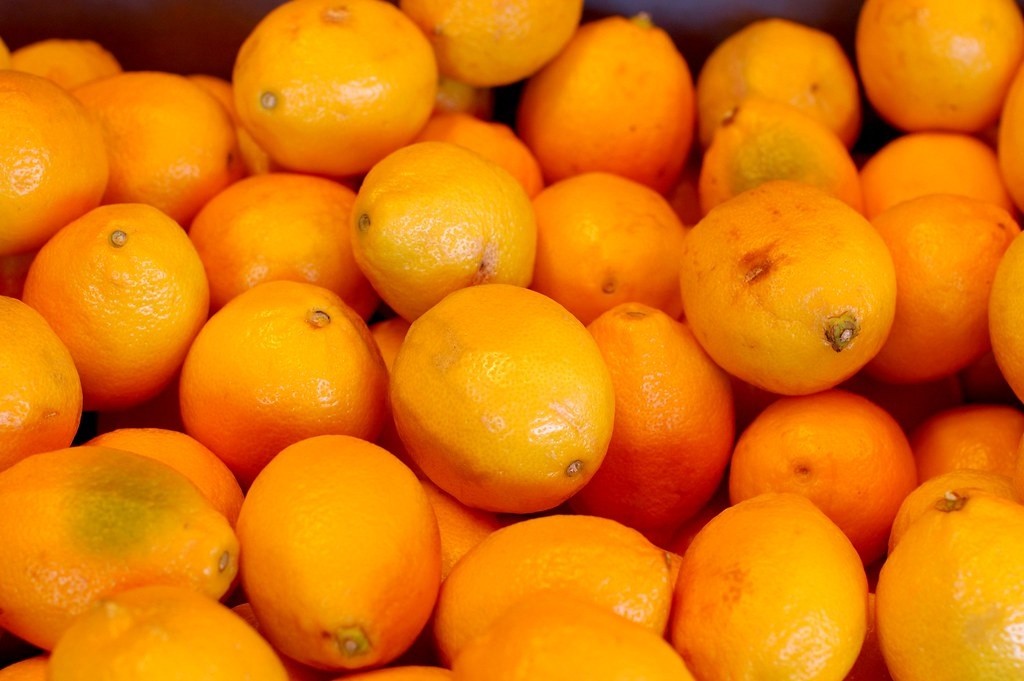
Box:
[0,0,1024,681]
